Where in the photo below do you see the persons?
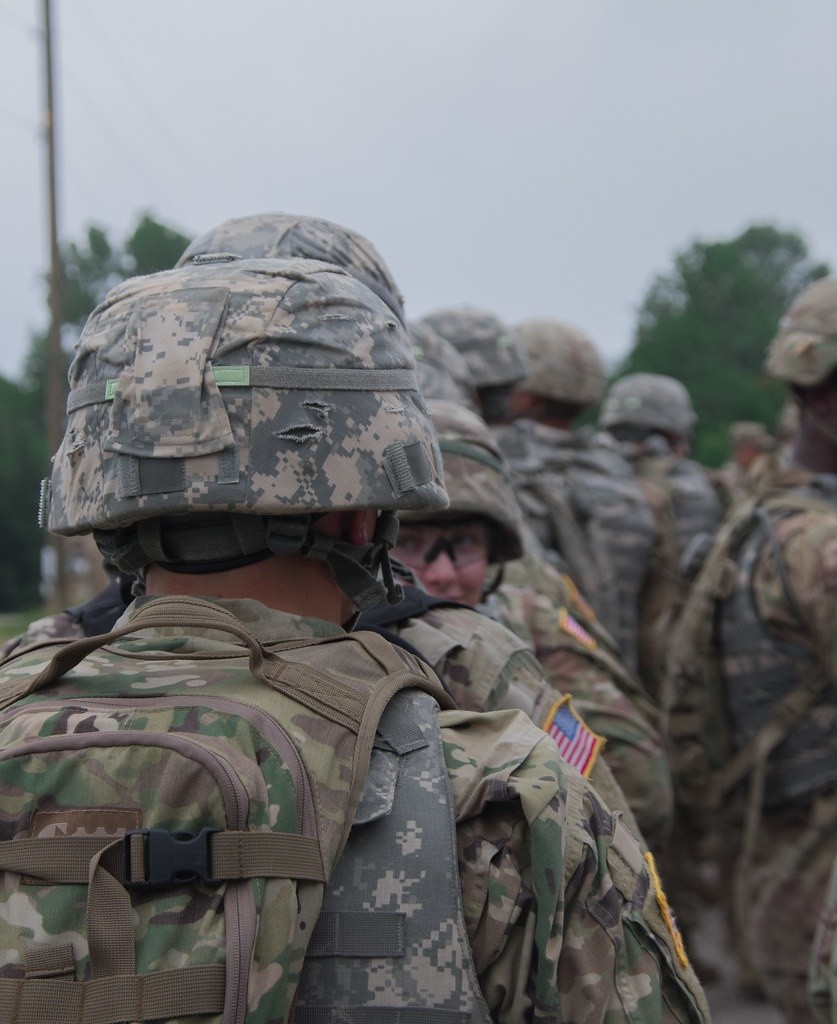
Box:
[413,301,806,699]
[376,397,676,885]
[168,216,652,865]
[0,255,718,1024]
[668,274,833,1017]
